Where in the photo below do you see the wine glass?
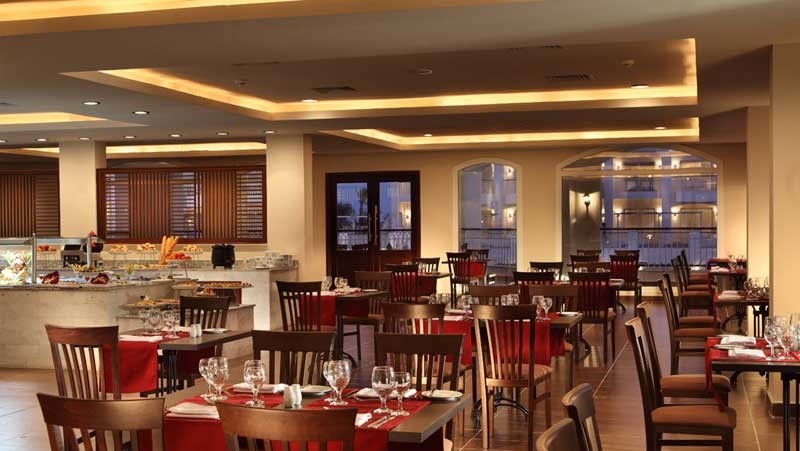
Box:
[139,305,181,339]
[743,276,769,299]
[198,355,411,417]
[427,292,552,322]
[527,267,611,282]
[323,275,351,293]
[764,311,800,361]
[727,245,746,273]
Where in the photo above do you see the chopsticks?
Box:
[367,414,397,430]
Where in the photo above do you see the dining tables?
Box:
[705,264,748,294]
[712,288,772,339]
[701,333,800,451]
[707,256,747,269]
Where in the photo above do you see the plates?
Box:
[421,390,462,401]
[362,289,378,292]
[167,407,220,415]
[202,328,228,333]
[556,312,579,317]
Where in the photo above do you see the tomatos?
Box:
[166,252,191,259]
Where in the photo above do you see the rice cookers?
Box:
[211,242,236,269]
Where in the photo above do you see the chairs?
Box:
[670,257,716,316]
[663,271,722,331]
[621,317,738,451]
[674,254,717,291]
[36,249,646,449]
[656,276,723,375]
[678,249,719,285]
[636,299,732,411]
